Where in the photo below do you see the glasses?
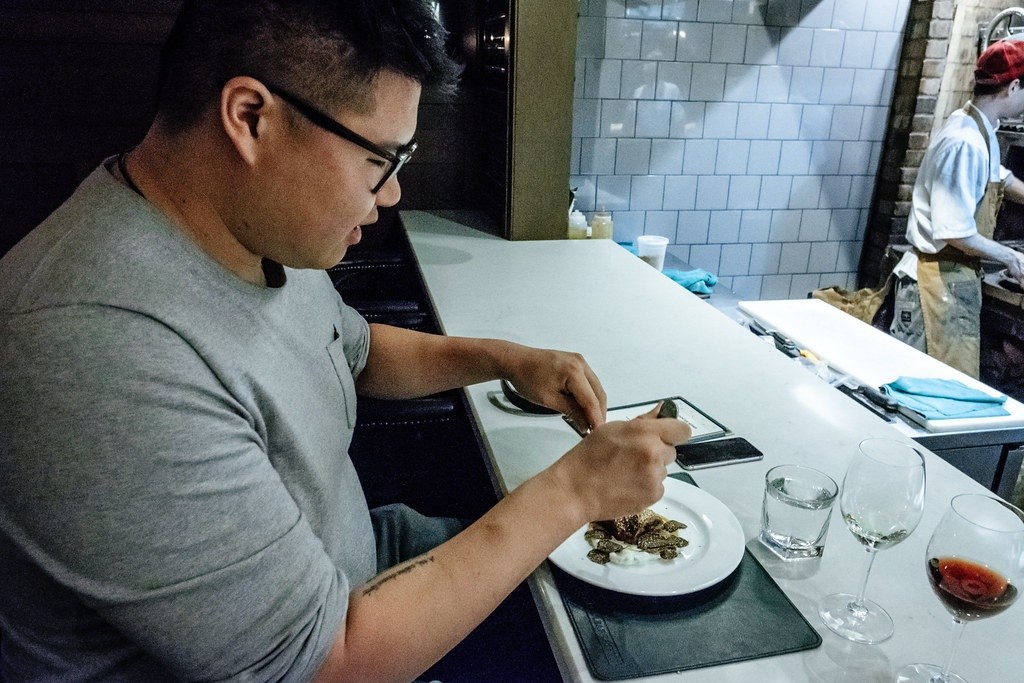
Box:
[217,69,419,194]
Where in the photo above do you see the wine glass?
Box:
[895,494,1023,683]
[818,431,926,644]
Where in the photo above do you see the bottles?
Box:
[569,209,587,239]
[592,206,612,239]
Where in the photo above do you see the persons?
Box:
[0,0,691,683]
[872,38,1024,380]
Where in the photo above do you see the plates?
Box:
[999,267,1019,285]
[548,477,746,597]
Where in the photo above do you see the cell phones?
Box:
[674,437,763,471]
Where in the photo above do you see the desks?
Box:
[892,241,1020,402]
[738,298,1019,429]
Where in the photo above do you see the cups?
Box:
[638,236,668,272]
[760,464,839,561]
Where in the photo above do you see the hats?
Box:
[975,33,1024,85]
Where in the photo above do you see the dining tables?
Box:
[400,207,1019,682]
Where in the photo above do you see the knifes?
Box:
[826,361,898,411]
[755,319,795,349]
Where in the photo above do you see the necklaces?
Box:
[117,146,145,197]
